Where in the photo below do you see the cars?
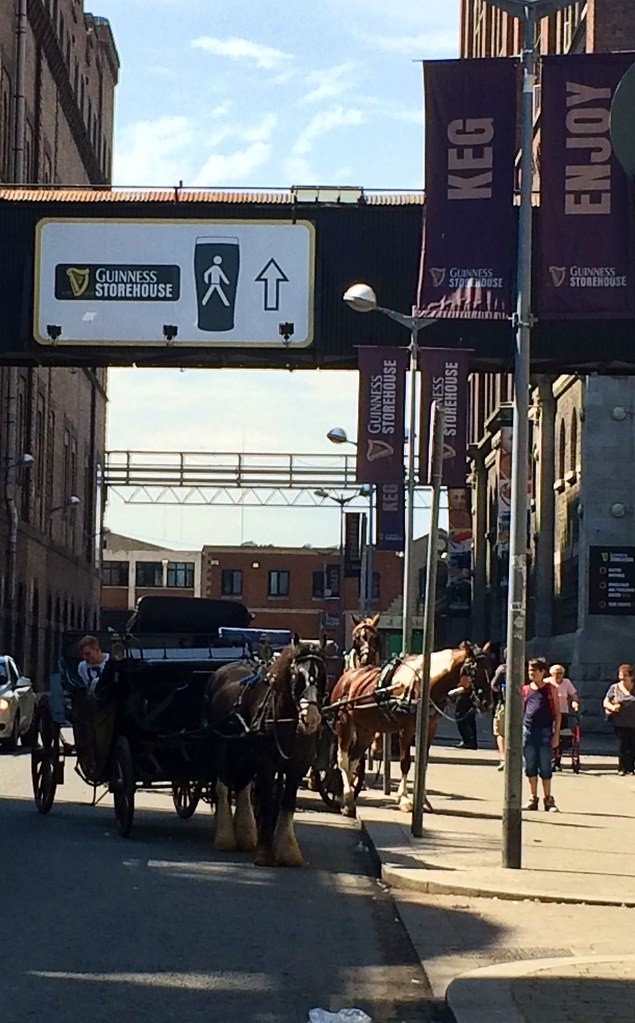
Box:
[0,655,39,753]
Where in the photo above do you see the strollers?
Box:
[553,708,582,774]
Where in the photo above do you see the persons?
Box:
[523,657,561,812]
[603,664,635,776]
[77,635,110,694]
[490,648,506,772]
[448,642,478,751]
[542,665,580,772]
[449,489,471,529]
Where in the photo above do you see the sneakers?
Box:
[522,796,539,810]
[544,796,559,812]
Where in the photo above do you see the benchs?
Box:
[125,633,257,664]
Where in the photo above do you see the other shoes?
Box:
[555,763,562,772]
[619,769,635,776]
[496,760,505,772]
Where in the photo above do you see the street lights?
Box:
[344,282,417,655]
[315,489,376,655]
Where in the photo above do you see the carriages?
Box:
[27,632,494,872]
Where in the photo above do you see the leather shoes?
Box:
[451,741,478,749]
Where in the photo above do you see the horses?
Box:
[201,630,328,872]
[330,611,497,821]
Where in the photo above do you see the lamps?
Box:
[278,322,294,347]
[163,325,178,349]
[46,325,61,344]
[52,496,83,514]
[0,453,35,472]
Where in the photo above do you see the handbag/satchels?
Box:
[604,683,620,715]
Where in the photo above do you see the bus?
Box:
[112,595,252,657]
